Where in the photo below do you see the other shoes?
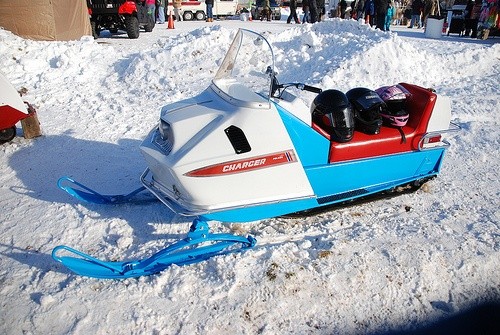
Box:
[159,22,164,24]
[408,26,412,28]
[206,18,214,22]
[296,22,300,24]
[287,22,292,24]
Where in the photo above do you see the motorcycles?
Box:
[140,29,463,223]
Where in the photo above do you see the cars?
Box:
[251,0,281,21]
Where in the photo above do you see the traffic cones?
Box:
[166,10,176,29]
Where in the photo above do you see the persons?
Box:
[337,0,393,32]
[286,0,325,25]
[259,0,273,21]
[205,0,214,22]
[455,0,500,41]
[145,0,166,24]
[407,0,439,29]
[172,0,183,22]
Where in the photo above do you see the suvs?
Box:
[86,0,156,39]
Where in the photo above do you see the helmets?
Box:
[311,89,354,142]
[347,88,386,135]
[375,85,411,127]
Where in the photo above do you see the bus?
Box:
[165,0,239,21]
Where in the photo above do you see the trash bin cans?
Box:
[425,15,445,38]
